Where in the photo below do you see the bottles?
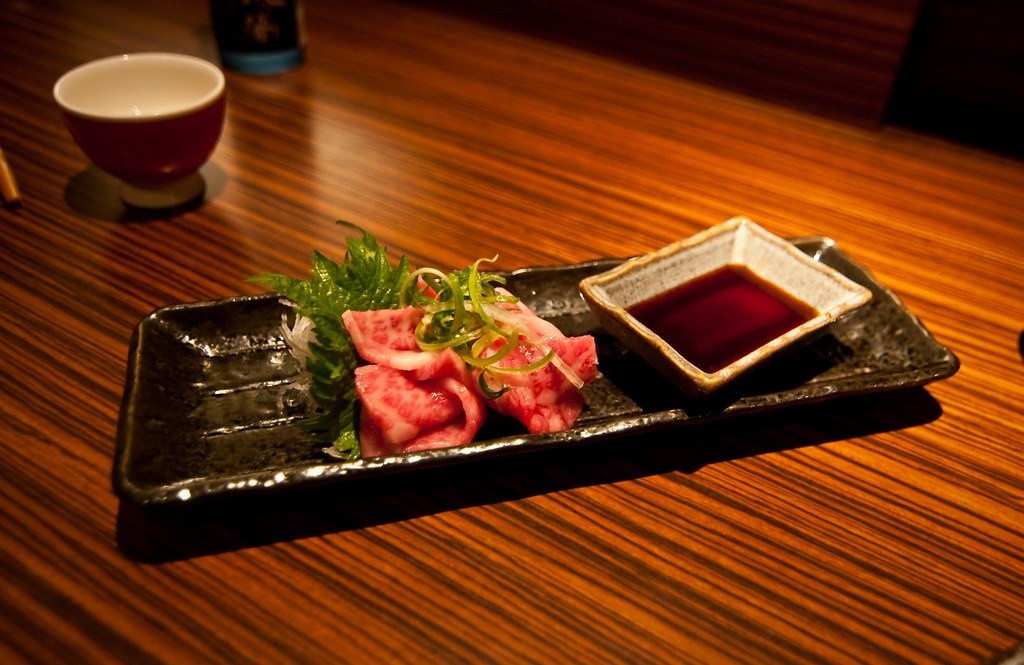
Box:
[209,1,307,77]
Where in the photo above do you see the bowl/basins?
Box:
[578,215,872,394]
[54,52,227,207]
[112,236,960,569]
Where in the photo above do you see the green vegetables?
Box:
[244,218,584,460]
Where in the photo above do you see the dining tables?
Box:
[0,0,1024,665]
